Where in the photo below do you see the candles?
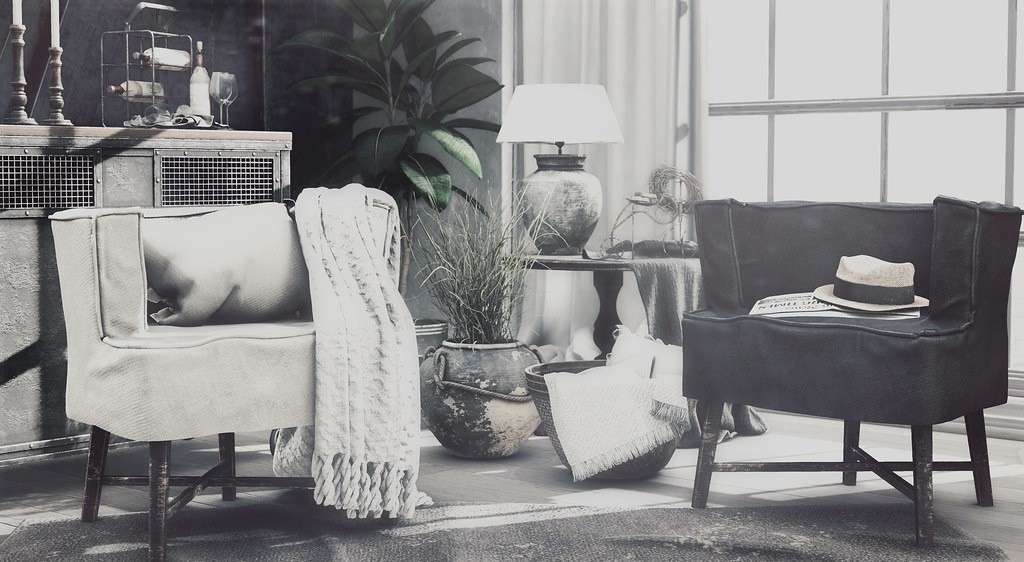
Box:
[12,0,22,25]
[50,0,60,47]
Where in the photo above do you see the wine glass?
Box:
[209,71,238,131]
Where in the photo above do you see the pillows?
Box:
[147,198,310,326]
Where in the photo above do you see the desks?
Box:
[499,254,702,360]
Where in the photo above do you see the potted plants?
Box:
[278,0,508,431]
[400,179,570,461]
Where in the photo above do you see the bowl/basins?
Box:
[524,359,683,479]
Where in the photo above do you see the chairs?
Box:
[48,183,434,562]
[681,193,1024,549]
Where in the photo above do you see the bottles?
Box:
[189,41,210,116]
[106,81,166,103]
[132,47,191,72]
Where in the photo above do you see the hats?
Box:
[814,255,929,311]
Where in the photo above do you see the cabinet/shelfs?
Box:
[0,124,294,471]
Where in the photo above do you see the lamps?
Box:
[495,84,624,256]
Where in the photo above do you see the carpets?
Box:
[0,501,1008,562]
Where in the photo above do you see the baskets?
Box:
[524,360,681,480]
